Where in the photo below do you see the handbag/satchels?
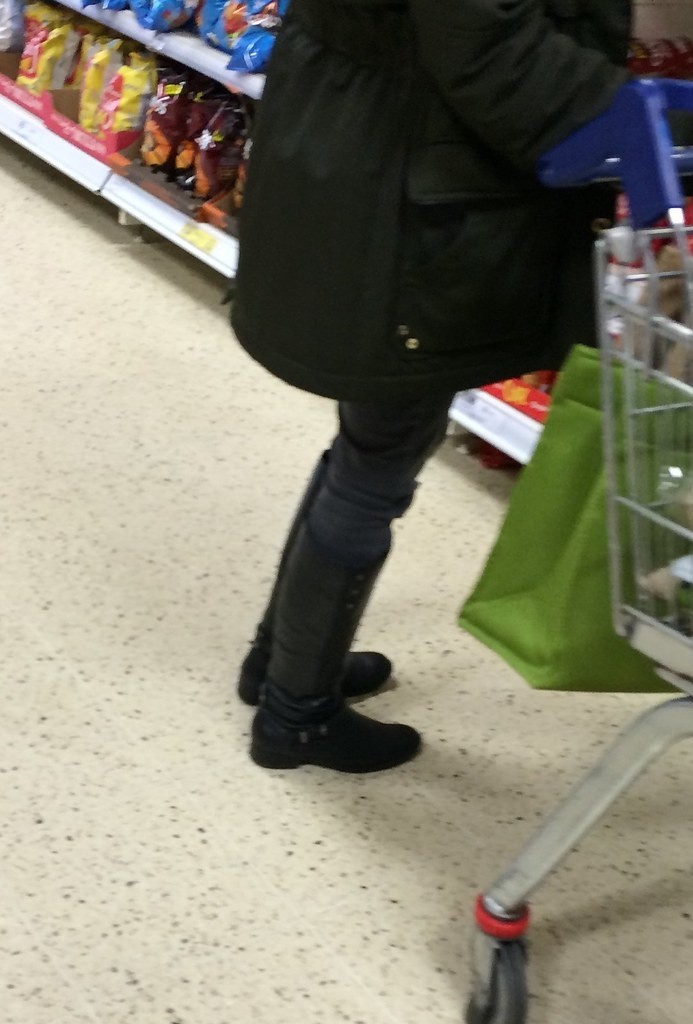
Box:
[457,347,693,691]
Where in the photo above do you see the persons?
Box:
[231,0,636,773]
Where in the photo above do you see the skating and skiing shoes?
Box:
[237,646,392,704]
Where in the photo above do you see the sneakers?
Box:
[248,699,420,773]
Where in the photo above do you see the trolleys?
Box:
[464,70,693,1024]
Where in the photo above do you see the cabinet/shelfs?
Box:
[0,1,549,467]
